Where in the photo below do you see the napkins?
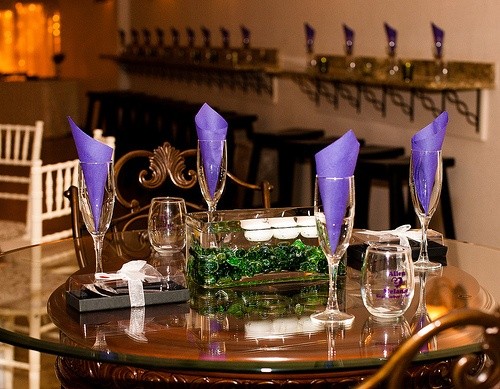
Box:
[411,110,448,214]
[315,128,360,253]
[195,102,228,198]
[118,19,447,58]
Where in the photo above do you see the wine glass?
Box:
[77,159,114,272]
[197,138,229,212]
[119,35,251,64]
[409,148,443,270]
[408,270,438,353]
[303,35,444,81]
[310,172,356,322]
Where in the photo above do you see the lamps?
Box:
[67,114,113,231]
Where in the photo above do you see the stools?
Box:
[83,87,457,242]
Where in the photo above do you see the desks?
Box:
[0,223,500,389]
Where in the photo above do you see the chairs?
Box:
[357,307,500,389]
[0,121,273,389]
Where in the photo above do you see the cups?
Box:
[358,315,412,359]
[317,56,330,73]
[191,48,202,62]
[361,245,415,319]
[401,61,416,81]
[363,56,374,77]
[209,48,218,62]
[148,196,188,254]
